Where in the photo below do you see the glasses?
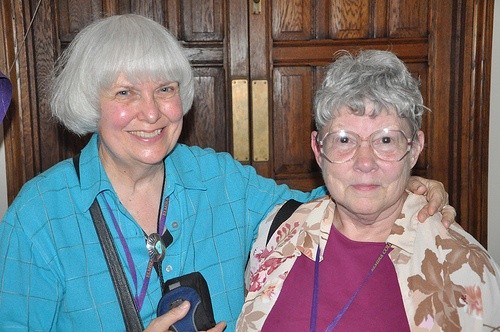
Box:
[315,128,419,164]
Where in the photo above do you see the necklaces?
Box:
[140,159,166,264]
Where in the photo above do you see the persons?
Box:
[235,49,500,332]
[1,14,463,332]
